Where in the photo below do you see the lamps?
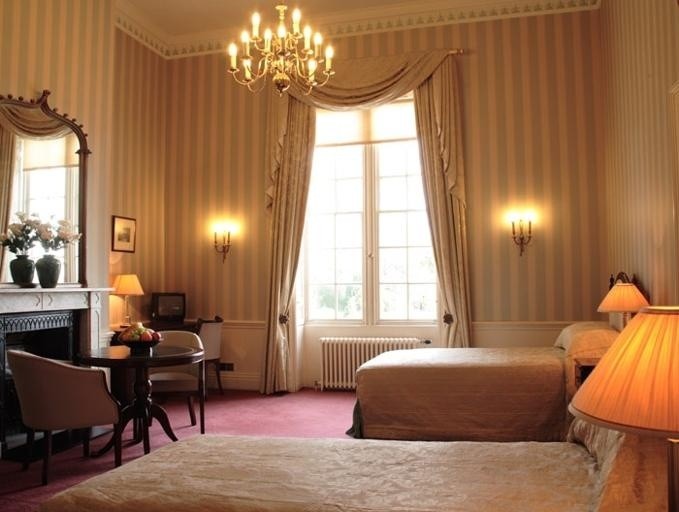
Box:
[505,207,534,256]
[227,0,336,95]
[110,274,144,324]
[567,306,679,512]
[597,284,649,328]
[211,217,239,263]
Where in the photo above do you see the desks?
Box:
[75,345,205,458]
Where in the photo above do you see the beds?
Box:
[39,416,679,512]
[356,272,638,441]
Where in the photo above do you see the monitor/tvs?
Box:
[152,292,185,323]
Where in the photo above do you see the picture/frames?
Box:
[112,215,136,253]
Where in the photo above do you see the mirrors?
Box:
[0,90,91,287]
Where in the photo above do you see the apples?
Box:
[119,322,161,342]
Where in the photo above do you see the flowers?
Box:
[0,212,40,255]
[27,216,80,255]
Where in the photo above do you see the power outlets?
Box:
[423,339,432,346]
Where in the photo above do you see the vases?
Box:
[11,256,34,284]
[35,255,61,288]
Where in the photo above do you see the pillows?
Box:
[554,321,619,358]
[568,411,673,512]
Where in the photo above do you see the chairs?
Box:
[110,317,224,439]
[7,350,122,487]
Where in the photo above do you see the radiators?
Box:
[319,337,418,393]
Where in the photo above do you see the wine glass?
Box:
[119,337,163,356]
